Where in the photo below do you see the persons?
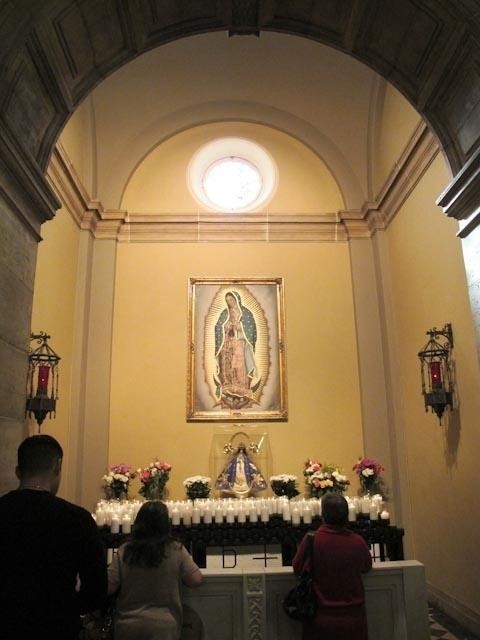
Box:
[2,434,108,640]
[107,501,203,640]
[293,492,371,639]
[215,287,258,409]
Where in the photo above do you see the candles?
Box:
[90,493,389,534]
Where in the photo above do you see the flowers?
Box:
[135,461,171,500]
[302,457,350,499]
[101,463,135,501]
[269,474,300,500]
[183,475,211,501]
[352,454,385,497]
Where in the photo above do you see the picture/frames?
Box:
[186,276,288,423]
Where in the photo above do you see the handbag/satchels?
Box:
[80,610,113,640]
[283,532,318,621]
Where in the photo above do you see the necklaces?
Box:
[19,483,45,491]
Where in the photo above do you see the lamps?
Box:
[418,324,453,426]
[25,331,61,433]
